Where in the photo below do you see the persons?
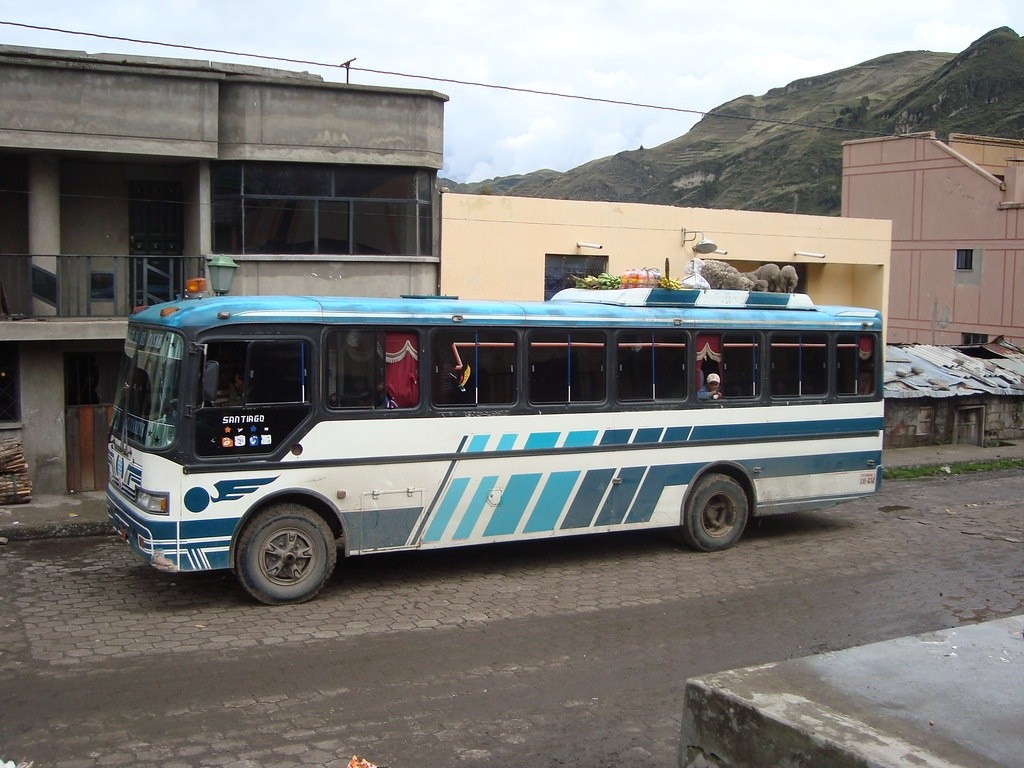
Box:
[697,373,722,400]
[225,370,271,406]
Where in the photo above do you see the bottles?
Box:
[621,268,660,289]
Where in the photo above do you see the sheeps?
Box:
[780,265,799,293]
[700,260,758,291]
[740,270,768,292]
[752,264,782,292]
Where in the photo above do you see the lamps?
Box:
[681,227,718,254]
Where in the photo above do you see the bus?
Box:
[105,286,886,607]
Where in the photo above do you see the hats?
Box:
[707,373,720,384]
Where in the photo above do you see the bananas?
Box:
[656,275,683,291]
[575,273,622,290]
